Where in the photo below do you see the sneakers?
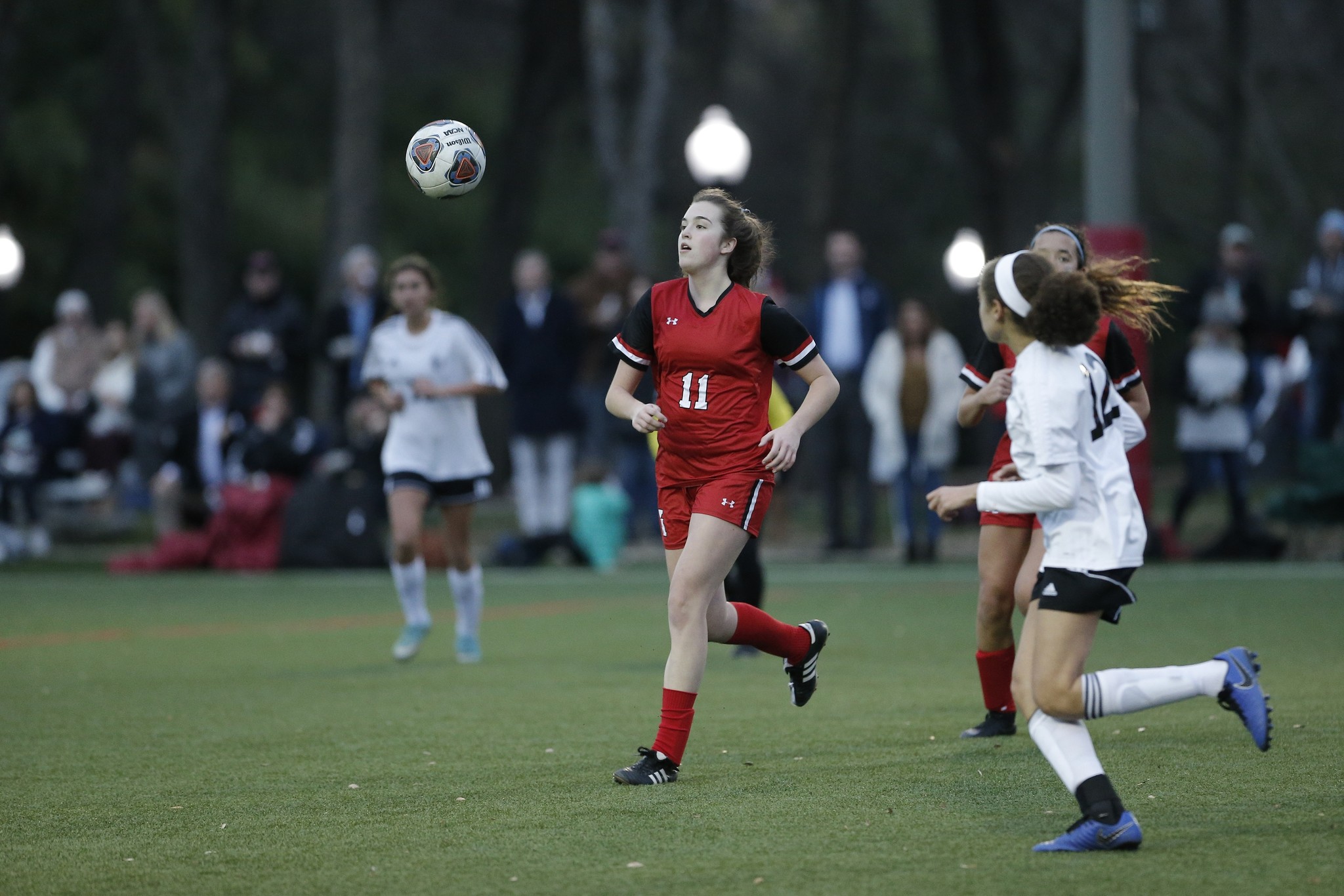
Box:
[612,746,679,786]
[963,710,1018,738]
[783,618,830,707]
[1212,647,1274,752]
[1034,810,1144,850]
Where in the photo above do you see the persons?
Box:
[926,252,1274,855]
[477,240,662,567]
[604,189,842,786]
[360,255,504,670]
[175,246,396,571]
[640,373,795,658]
[781,224,890,555]
[859,296,973,570]
[955,224,1150,737]
[0,285,241,557]
[1155,200,1344,563]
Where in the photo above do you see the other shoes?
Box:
[732,641,758,658]
[394,614,431,660]
[453,619,484,665]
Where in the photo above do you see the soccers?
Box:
[406,119,487,200]
